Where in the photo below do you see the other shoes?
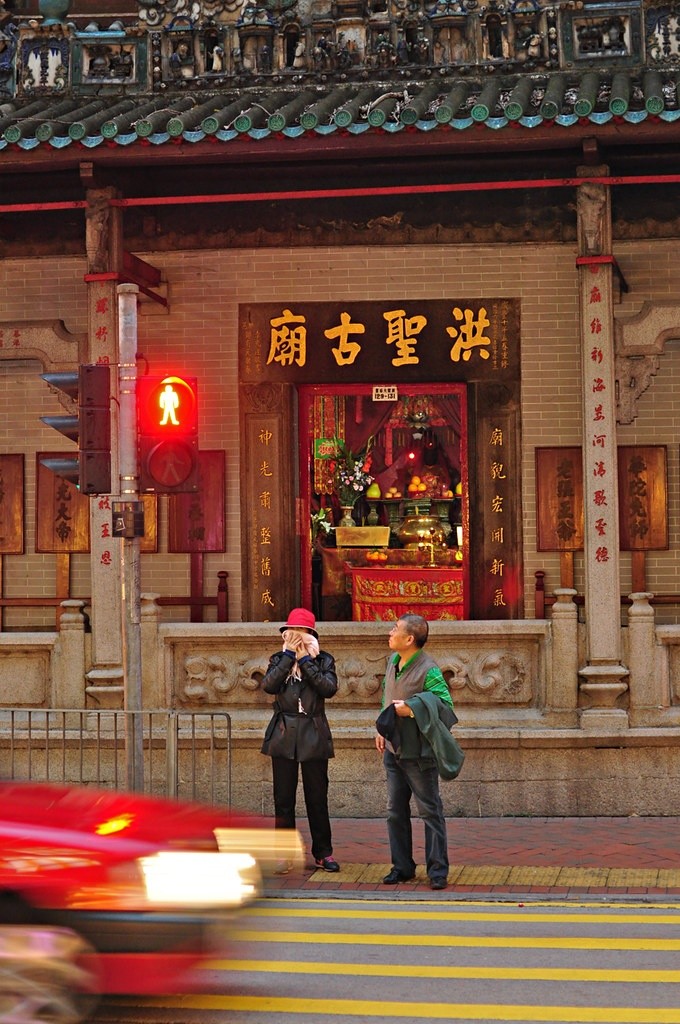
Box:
[430,876,447,889]
[383,868,404,884]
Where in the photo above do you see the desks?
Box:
[317,542,463,621]
[344,562,463,622]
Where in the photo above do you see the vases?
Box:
[311,547,314,556]
[339,506,356,528]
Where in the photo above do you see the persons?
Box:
[375,613,456,890]
[260,608,340,874]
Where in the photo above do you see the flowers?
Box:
[324,448,375,507]
[309,508,335,547]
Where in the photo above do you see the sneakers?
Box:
[314,855,340,871]
[273,858,294,874]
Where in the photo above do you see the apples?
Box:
[384,487,401,498]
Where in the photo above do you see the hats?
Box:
[279,608,319,640]
[375,703,401,753]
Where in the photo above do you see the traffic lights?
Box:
[135,377,199,493]
[39,363,112,491]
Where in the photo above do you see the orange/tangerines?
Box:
[408,476,426,491]
[366,551,387,559]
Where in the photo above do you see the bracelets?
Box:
[410,710,415,718]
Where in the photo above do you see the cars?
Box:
[0,773,306,1024]
